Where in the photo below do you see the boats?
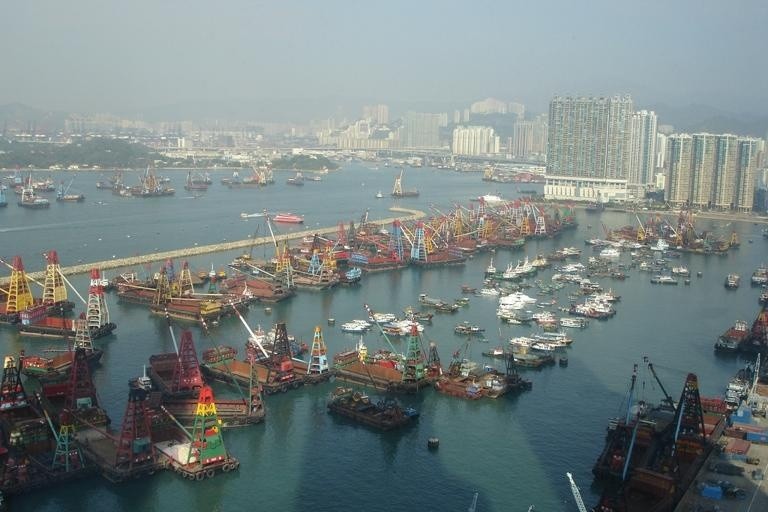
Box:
[390,169,421,198]
